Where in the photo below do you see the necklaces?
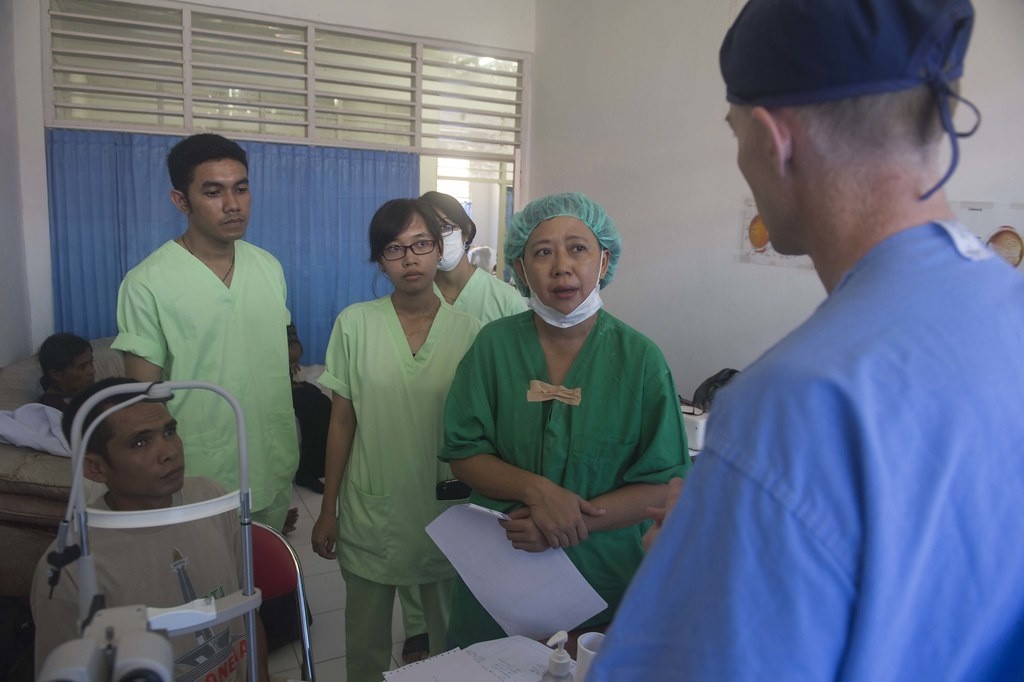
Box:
[180,231,235,283]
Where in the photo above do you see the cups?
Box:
[576,632,606,682]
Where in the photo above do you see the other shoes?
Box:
[295,469,325,493]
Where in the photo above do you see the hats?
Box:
[720,0,982,201]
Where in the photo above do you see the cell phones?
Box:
[436,479,471,500]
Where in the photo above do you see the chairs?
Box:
[249,521,313,681]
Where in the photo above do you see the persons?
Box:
[583,0,1024,682]
[285,323,335,494]
[312,196,534,682]
[399,192,529,662]
[109,133,299,537]
[29,376,260,682]
[33,333,299,537]
[440,194,691,654]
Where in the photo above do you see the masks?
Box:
[519,250,604,329]
[437,229,466,271]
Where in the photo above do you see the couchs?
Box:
[1,331,124,603]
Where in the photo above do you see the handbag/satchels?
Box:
[694,368,740,416]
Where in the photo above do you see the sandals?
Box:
[402,632,430,656]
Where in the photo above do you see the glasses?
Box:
[439,223,460,237]
[381,238,438,261]
